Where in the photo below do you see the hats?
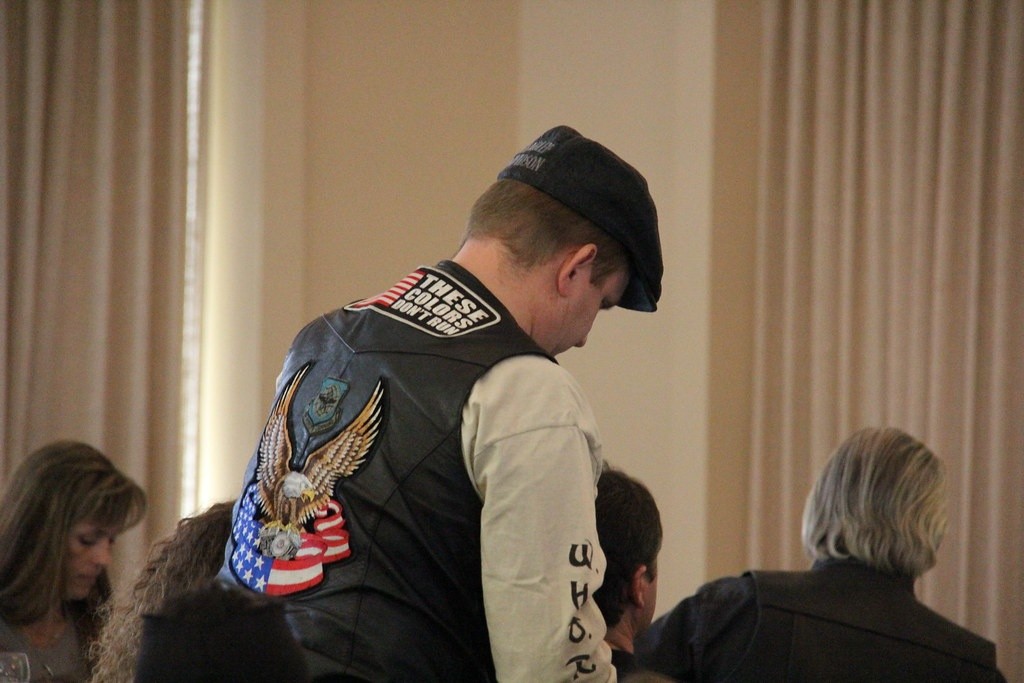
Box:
[497,125,664,312]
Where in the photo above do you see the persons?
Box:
[594,470,674,683]
[93,126,664,683]
[639,427,1007,683]
[0,439,147,683]
